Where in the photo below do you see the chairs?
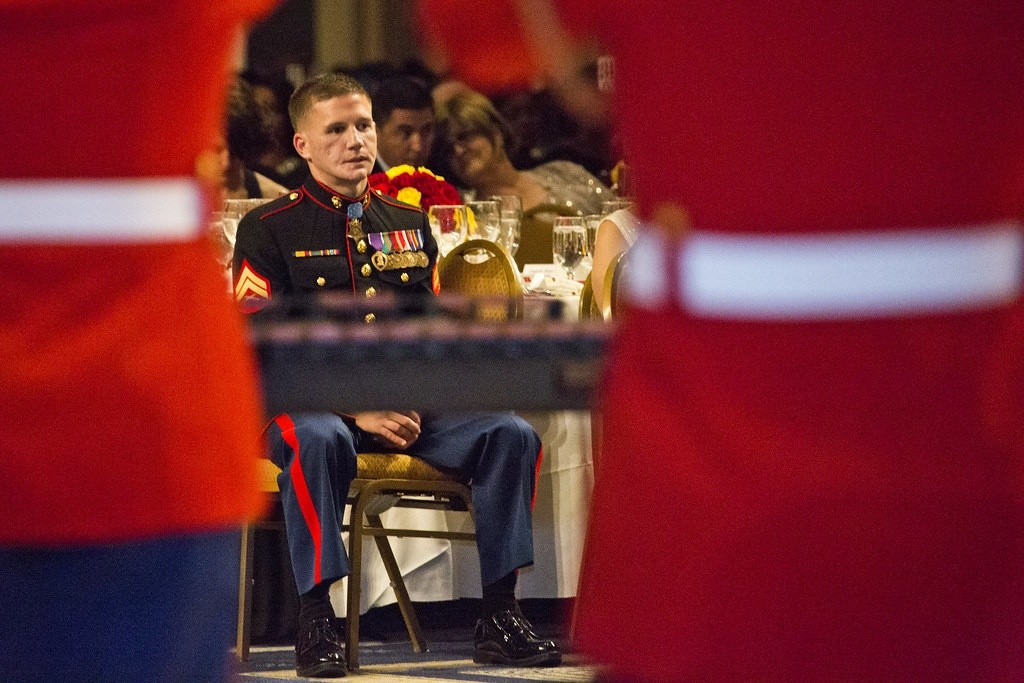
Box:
[603,249,630,324]
[512,201,587,272]
[235,239,524,673]
[577,269,603,481]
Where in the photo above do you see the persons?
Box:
[221,55,627,220]
[1,0,283,683]
[415,1,1024,683]
[232,74,561,678]
[592,202,644,325]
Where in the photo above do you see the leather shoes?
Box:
[467,607,561,664]
[293,607,349,675]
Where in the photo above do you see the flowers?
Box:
[365,164,476,234]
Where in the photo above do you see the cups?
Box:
[223,199,275,248]
[586,215,606,260]
[428,195,522,260]
[600,200,633,215]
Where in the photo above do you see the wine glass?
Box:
[206,211,235,273]
[552,216,588,281]
